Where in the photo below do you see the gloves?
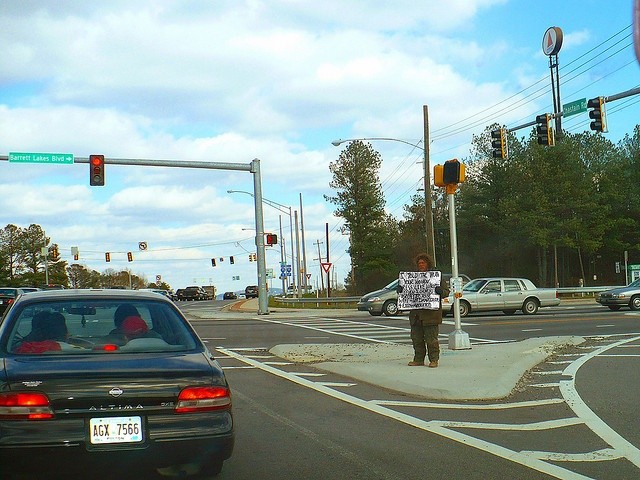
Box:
[398,285,403,295]
[436,286,443,295]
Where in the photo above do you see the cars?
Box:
[441,276,561,317]
[596,278,640,310]
[223,292,237,298]
[1,289,234,480]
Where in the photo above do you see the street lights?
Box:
[227,190,295,298]
[331,136,435,266]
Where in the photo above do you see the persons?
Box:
[396,253,450,368]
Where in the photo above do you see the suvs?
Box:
[176,289,186,301]
[245,286,259,299]
[182,286,207,300]
[357,274,471,316]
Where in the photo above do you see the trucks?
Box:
[202,286,217,300]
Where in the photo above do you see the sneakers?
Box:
[408,361,424,366]
[429,361,439,367]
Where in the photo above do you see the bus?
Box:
[287,283,314,295]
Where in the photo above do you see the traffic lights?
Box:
[490,128,505,160]
[536,113,551,147]
[212,259,216,267]
[89,154,105,186]
[74,254,78,260]
[127,252,133,262]
[253,253,257,262]
[267,235,278,244]
[230,256,234,264]
[106,252,110,262]
[249,254,253,261]
[588,96,605,132]
[443,159,460,186]
[52,249,57,257]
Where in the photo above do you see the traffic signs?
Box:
[8,152,75,165]
[562,98,586,118]
